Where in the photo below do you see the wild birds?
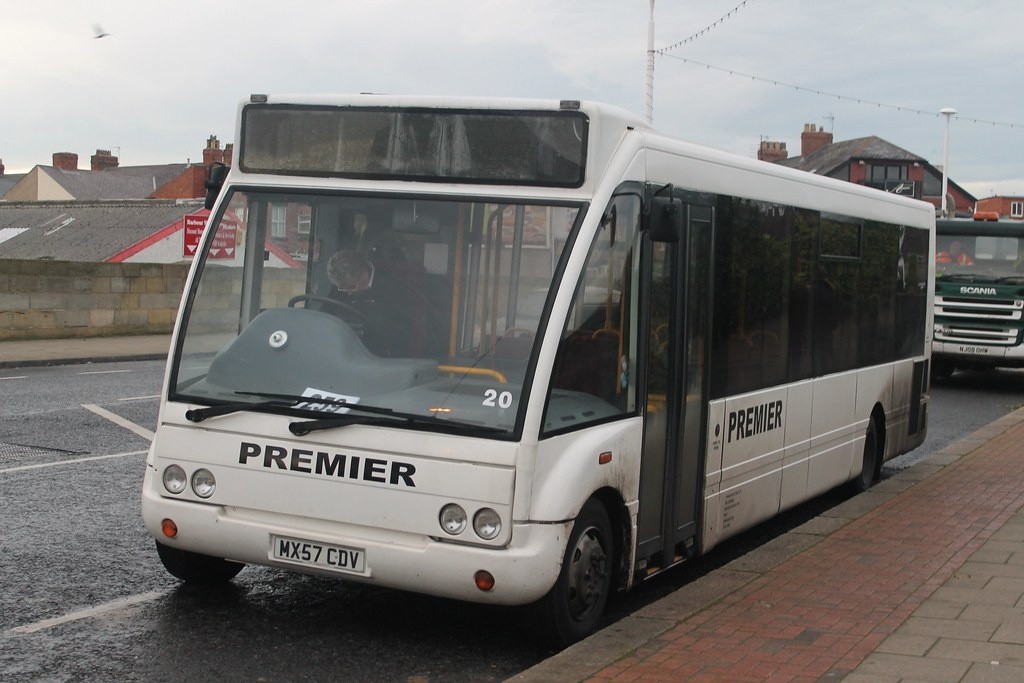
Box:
[90,22,111,39]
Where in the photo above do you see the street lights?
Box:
[939,107,958,213]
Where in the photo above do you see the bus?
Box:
[139,94,937,641]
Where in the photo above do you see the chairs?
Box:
[485,329,619,398]
[344,238,432,357]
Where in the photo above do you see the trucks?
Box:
[931,211,1024,385]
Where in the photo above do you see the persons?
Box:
[936,239,976,266]
[317,248,438,357]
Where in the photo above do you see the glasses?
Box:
[337,281,362,292]
